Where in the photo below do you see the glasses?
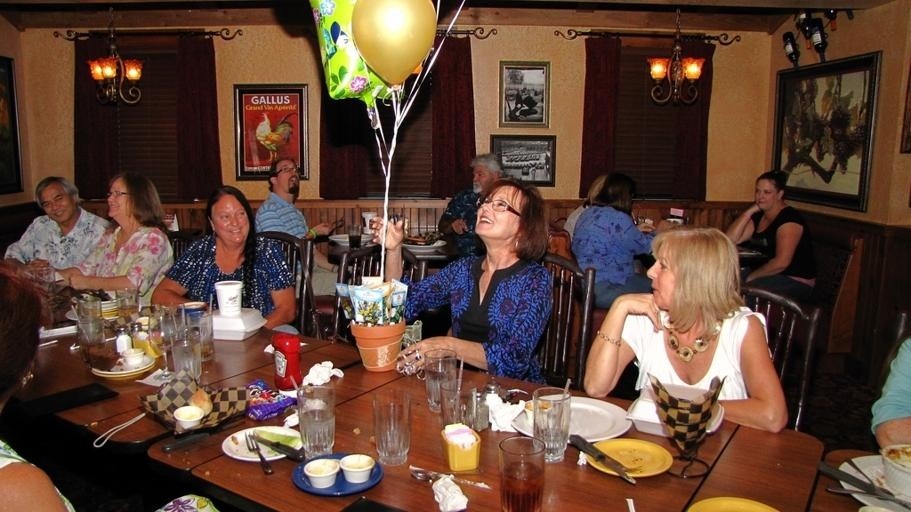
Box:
[273,166,302,176]
[106,190,129,197]
[476,197,524,219]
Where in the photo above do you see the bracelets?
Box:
[309,229,317,239]
[596,331,621,347]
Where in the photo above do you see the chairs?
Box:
[769,232,866,366]
[525,253,596,388]
[330,246,430,350]
[544,229,609,377]
[252,232,314,335]
[729,287,822,432]
[169,231,205,265]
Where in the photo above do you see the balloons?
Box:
[309,0,403,101]
[351,0,437,94]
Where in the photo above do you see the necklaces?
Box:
[663,315,721,362]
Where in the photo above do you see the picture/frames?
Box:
[498,59,552,130]
[0,55,25,197]
[771,49,882,210]
[232,82,310,182]
[490,134,558,189]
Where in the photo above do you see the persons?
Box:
[871,336,911,450]
[370,179,554,389]
[563,174,607,244]
[150,184,301,336]
[31,171,175,308]
[3,175,111,282]
[570,172,656,309]
[438,154,502,263]
[0,254,220,512]
[584,227,789,434]
[253,157,362,299]
[725,170,816,297]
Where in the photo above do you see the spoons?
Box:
[408,464,491,491]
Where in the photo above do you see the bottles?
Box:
[467,390,489,431]
[482,381,501,402]
[131,323,142,339]
[116,325,132,353]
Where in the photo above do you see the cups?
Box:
[441,381,477,427]
[440,425,481,472]
[362,212,377,235]
[214,281,244,314]
[296,386,336,458]
[372,387,413,466]
[115,288,140,315]
[498,436,546,511]
[423,349,456,415]
[340,453,375,483]
[185,311,214,362]
[173,406,205,429]
[165,327,203,384]
[80,298,102,319]
[124,348,146,367]
[534,386,570,463]
[77,320,101,362]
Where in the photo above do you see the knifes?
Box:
[571,435,638,485]
[161,418,245,454]
[249,433,305,463]
[820,463,896,501]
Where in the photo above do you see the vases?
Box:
[347,319,406,372]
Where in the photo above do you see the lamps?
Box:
[85,7,142,107]
[646,11,704,106]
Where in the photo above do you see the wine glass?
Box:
[149,304,176,382]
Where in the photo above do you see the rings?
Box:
[654,311,659,315]
[416,353,421,360]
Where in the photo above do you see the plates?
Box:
[91,356,155,383]
[221,425,303,461]
[585,437,674,477]
[40,324,79,336]
[510,396,631,442]
[63,293,122,328]
[839,454,911,511]
[292,454,382,496]
[688,497,778,512]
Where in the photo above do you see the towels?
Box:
[647,370,727,456]
[133,366,249,438]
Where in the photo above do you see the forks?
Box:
[247,433,275,474]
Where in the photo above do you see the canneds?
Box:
[272,324,303,389]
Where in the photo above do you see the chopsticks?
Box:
[70,336,117,350]
[40,340,58,347]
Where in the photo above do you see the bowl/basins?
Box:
[400,240,448,256]
[882,442,911,502]
[328,233,374,247]
[182,301,206,319]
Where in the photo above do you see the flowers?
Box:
[342,297,402,326]
